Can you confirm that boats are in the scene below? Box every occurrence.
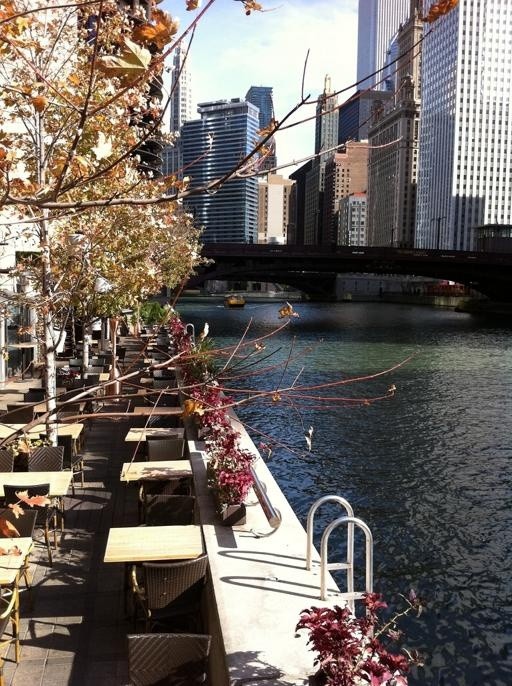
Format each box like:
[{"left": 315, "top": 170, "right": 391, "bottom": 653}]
[{"left": 223, "top": 297, "right": 246, "bottom": 308}]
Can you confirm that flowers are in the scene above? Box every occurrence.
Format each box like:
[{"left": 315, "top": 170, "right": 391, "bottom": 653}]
[
  {"left": 295, "top": 591, "right": 424, "bottom": 686},
  {"left": 193, "top": 381, "right": 257, "bottom": 505}
]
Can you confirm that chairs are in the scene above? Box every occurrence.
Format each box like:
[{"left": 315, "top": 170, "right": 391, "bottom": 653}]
[{"left": 0, "top": 329, "right": 212, "bottom": 686}]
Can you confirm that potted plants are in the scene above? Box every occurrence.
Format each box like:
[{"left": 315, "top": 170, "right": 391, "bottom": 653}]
[{"left": 120, "top": 314, "right": 129, "bottom": 337}]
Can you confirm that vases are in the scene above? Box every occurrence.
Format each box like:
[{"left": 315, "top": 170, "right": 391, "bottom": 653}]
[{"left": 211, "top": 486, "right": 246, "bottom": 525}]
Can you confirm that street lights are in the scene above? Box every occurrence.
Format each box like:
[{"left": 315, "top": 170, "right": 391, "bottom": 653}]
[
  {"left": 384, "top": 224, "right": 401, "bottom": 247},
  {"left": 431, "top": 216, "right": 447, "bottom": 249}
]
